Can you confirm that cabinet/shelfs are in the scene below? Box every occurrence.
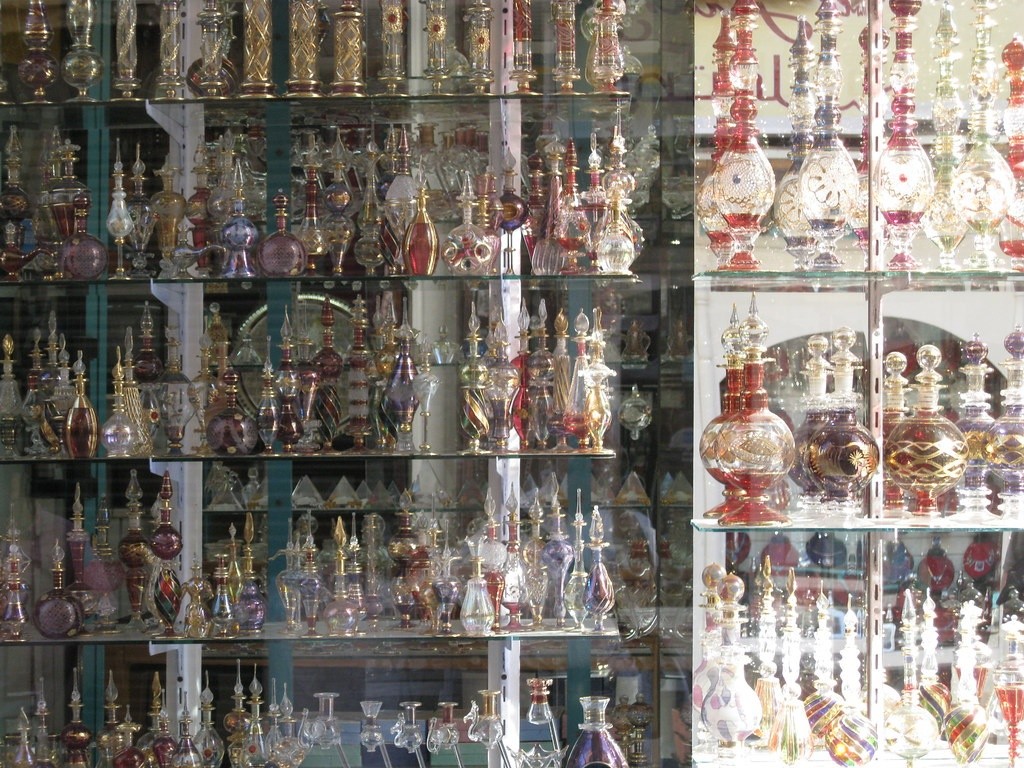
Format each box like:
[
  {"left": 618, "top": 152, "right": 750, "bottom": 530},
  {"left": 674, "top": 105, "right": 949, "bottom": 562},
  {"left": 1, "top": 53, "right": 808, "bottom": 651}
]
[{"left": 0, "top": 0, "right": 1024, "bottom": 768}]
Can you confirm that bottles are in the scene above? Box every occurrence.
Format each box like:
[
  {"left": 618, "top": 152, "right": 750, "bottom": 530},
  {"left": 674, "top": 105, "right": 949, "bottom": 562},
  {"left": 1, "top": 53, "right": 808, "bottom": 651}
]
[{"left": 0, "top": 1, "right": 1024, "bottom": 766}]
